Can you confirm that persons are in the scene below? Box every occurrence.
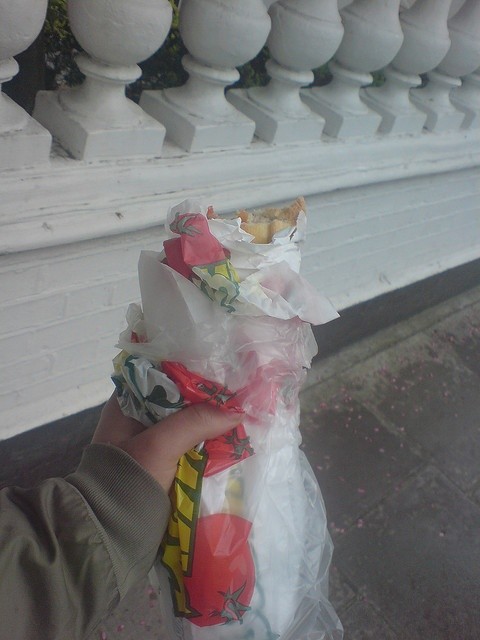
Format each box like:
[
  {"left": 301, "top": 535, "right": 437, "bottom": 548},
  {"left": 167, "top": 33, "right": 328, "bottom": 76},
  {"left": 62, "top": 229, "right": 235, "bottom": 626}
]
[{"left": 1, "top": 387, "right": 247, "bottom": 639}]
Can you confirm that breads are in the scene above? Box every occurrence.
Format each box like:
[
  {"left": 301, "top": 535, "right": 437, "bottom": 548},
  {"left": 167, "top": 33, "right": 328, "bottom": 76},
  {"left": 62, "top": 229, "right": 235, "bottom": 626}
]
[{"left": 207, "top": 194, "right": 308, "bottom": 243}]
